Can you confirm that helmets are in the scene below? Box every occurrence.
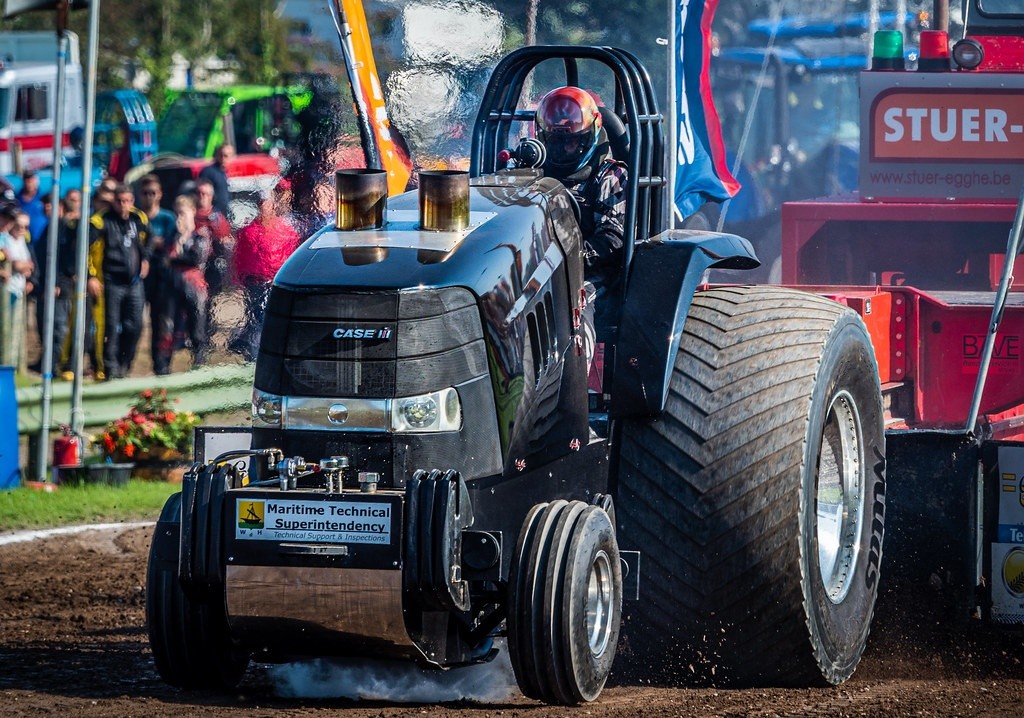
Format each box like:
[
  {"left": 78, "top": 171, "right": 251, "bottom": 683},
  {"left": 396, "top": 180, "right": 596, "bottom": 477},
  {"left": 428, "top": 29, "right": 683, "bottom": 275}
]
[{"left": 534, "top": 86, "right": 602, "bottom": 177}]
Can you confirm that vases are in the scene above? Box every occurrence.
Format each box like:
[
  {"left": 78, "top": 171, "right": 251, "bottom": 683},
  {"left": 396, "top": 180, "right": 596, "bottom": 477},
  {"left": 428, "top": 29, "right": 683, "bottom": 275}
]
[
  {"left": 60, "top": 460, "right": 132, "bottom": 486},
  {"left": 131, "top": 447, "right": 188, "bottom": 483}
]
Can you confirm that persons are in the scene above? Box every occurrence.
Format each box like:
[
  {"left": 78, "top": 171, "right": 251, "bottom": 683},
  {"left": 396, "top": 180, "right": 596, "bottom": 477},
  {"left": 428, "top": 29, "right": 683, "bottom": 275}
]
[
  {"left": 0, "top": 142, "right": 337, "bottom": 382},
  {"left": 535, "top": 86, "right": 629, "bottom": 378}
]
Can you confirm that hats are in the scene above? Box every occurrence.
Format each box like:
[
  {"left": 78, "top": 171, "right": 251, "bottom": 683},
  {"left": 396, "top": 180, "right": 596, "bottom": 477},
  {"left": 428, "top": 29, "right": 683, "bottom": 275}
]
[
  {"left": 114, "top": 185, "right": 133, "bottom": 193},
  {"left": 141, "top": 174, "right": 160, "bottom": 184},
  {"left": 275, "top": 179, "right": 292, "bottom": 194}
]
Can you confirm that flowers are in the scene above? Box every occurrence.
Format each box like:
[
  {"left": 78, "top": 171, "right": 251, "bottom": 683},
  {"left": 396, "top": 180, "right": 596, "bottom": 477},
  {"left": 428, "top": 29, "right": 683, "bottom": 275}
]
[{"left": 95, "top": 390, "right": 201, "bottom": 465}]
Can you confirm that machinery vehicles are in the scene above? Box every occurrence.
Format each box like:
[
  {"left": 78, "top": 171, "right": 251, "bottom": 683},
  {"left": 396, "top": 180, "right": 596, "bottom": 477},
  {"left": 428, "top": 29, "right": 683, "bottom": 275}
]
[
  {"left": 144, "top": 47, "right": 886, "bottom": 709},
  {"left": 0, "top": 1, "right": 1024, "bottom": 450}
]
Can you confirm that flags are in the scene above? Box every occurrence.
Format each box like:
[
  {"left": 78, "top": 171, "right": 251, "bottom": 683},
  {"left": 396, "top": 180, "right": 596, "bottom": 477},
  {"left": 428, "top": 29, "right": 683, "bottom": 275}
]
[{"left": 670, "top": 0, "right": 741, "bottom": 223}]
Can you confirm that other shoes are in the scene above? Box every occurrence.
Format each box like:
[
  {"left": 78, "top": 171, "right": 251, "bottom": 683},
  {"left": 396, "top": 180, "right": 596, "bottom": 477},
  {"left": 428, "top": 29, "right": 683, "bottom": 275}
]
[
  {"left": 96, "top": 371, "right": 105, "bottom": 379},
  {"left": 61, "top": 370, "right": 74, "bottom": 380}
]
